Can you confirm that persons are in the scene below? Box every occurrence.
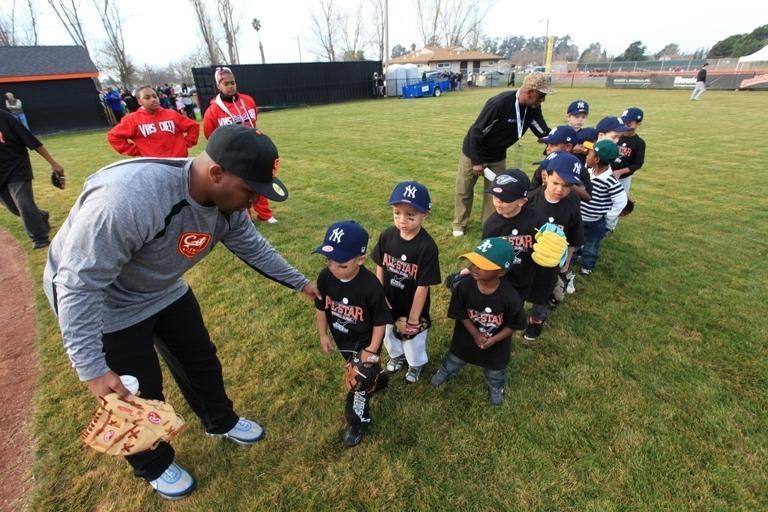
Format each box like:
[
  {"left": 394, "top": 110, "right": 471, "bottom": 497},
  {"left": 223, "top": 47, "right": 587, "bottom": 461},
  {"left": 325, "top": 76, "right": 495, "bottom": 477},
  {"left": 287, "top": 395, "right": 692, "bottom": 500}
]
[
  {"left": 507, "top": 65, "right": 515, "bottom": 87},
  {"left": 376, "top": 75, "right": 384, "bottom": 98},
  {"left": 690, "top": 63, "right": 709, "bottom": 101},
  {"left": 40, "top": 123, "right": 325, "bottom": 503},
  {"left": 572, "top": 126, "right": 598, "bottom": 155},
  {"left": 610, "top": 107, "right": 645, "bottom": 193},
  {"left": 104, "top": 85, "right": 200, "bottom": 158},
  {"left": 445, "top": 169, "right": 570, "bottom": 306},
  {"left": 200, "top": 66, "right": 279, "bottom": 224},
  {"left": 529, "top": 125, "right": 593, "bottom": 203},
  {"left": 428, "top": 238, "right": 529, "bottom": 409},
  {"left": 543, "top": 98, "right": 598, "bottom": 165},
  {"left": 421, "top": 68, "right": 473, "bottom": 92},
  {"left": 4, "top": 92, "right": 30, "bottom": 133},
  {"left": 308, "top": 218, "right": 391, "bottom": 448},
  {"left": 597, "top": 116, "right": 634, "bottom": 144},
  {"left": 0, "top": 108, "right": 67, "bottom": 250},
  {"left": 570, "top": 139, "right": 628, "bottom": 275},
  {"left": 367, "top": 180, "right": 443, "bottom": 386},
  {"left": 451, "top": 71, "right": 561, "bottom": 237},
  {"left": 103, "top": 83, "right": 198, "bottom": 123},
  {"left": 524, "top": 151, "right": 586, "bottom": 342}
]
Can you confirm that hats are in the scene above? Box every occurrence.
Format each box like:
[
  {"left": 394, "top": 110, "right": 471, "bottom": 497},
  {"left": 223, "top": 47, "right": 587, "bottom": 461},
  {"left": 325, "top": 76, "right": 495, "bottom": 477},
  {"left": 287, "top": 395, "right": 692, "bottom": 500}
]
[
  {"left": 457, "top": 237, "right": 516, "bottom": 272},
  {"left": 483, "top": 169, "right": 530, "bottom": 203},
  {"left": 387, "top": 182, "right": 432, "bottom": 212},
  {"left": 523, "top": 71, "right": 558, "bottom": 95},
  {"left": 311, "top": 221, "right": 369, "bottom": 264},
  {"left": 205, "top": 124, "right": 288, "bottom": 201},
  {"left": 533, "top": 100, "right": 643, "bottom": 184}
]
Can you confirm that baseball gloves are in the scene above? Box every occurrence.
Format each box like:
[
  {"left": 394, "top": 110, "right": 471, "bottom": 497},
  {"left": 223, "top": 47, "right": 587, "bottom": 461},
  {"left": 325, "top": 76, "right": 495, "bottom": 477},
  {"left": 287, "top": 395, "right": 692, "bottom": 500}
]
[
  {"left": 532, "top": 223, "right": 568, "bottom": 269},
  {"left": 82, "top": 393, "right": 184, "bottom": 456}
]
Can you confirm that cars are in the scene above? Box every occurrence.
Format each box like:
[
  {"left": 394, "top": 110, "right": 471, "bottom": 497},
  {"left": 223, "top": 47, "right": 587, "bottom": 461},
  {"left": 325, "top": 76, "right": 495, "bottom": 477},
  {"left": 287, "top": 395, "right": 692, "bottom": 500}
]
[{"left": 524, "top": 66, "right": 545, "bottom": 74}]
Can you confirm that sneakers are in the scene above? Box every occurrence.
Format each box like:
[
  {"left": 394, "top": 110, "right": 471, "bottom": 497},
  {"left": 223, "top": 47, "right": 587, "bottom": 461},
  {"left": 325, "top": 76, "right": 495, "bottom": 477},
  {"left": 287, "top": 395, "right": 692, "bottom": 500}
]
[
  {"left": 32, "top": 210, "right": 49, "bottom": 248},
  {"left": 149, "top": 461, "right": 197, "bottom": 501},
  {"left": 452, "top": 230, "right": 464, "bottom": 237},
  {"left": 386, "top": 353, "right": 449, "bottom": 388},
  {"left": 205, "top": 416, "right": 264, "bottom": 444},
  {"left": 267, "top": 216, "right": 278, "bottom": 224},
  {"left": 489, "top": 385, "right": 503, "bottom": 405},
  {"left": 523, "top": 256, "right": 592, "bottom": 341},
  {"left": 344, "top": 423, "right": 368, "bottom": 445}
]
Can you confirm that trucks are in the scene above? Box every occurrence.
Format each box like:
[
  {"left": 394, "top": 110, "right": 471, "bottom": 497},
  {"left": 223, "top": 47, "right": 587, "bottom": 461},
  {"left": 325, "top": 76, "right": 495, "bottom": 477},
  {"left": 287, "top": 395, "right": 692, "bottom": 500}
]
[{"left": 402, "top": 69, "right": 461, "bottom": 99}]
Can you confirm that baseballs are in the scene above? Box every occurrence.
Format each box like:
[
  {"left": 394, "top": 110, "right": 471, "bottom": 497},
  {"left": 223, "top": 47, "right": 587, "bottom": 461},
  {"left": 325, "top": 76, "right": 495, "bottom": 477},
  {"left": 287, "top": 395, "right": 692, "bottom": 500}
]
[{"left": 119, "top": 375, "right": 139, "bottom": 394}]
